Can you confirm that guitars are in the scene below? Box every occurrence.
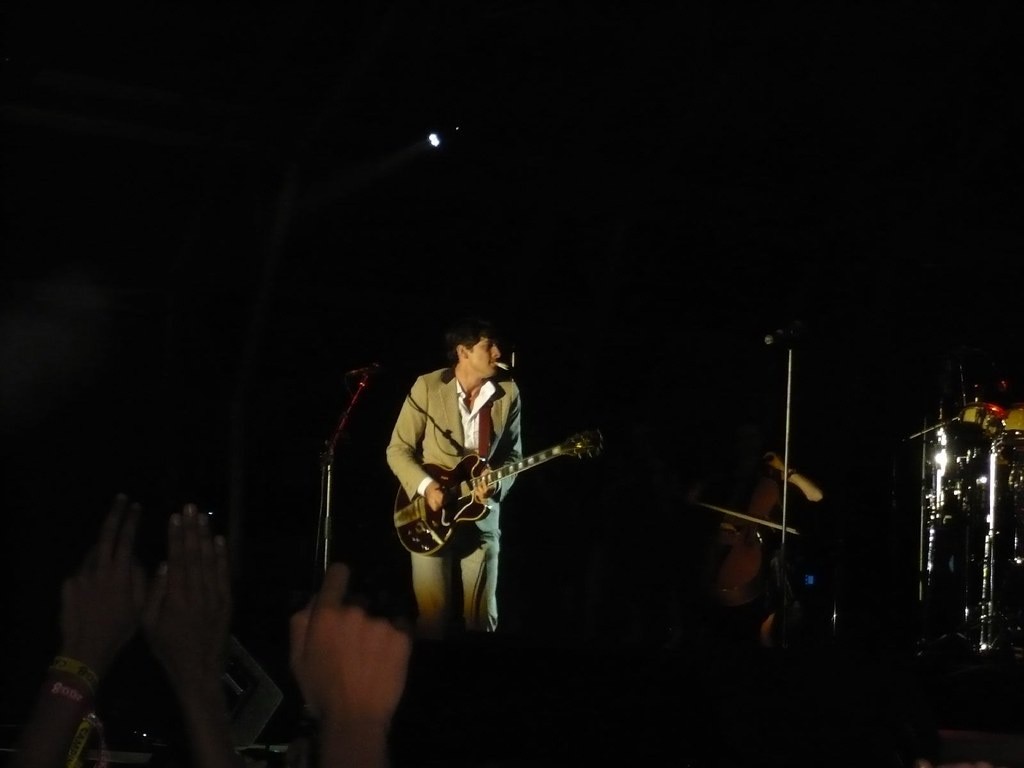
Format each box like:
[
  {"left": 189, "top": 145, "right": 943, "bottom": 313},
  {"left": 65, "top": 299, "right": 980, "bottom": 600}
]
[{"left": 393, "top": 428, "right": 606, "bottom": 557}]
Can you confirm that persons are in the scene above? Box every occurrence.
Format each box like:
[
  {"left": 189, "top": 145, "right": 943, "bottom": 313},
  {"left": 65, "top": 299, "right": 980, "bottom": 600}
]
[
  {"left": 387, "top": 313, "right": 524, "bottom": 635},
  {"left": 16, "top": 489, "right": 413, "bottom": 768},
  {"left": 689, "top": 417, "right": 823, "bottom": 582}
]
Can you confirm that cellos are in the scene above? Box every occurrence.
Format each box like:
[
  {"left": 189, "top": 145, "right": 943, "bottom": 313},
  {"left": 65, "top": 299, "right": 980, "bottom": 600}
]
[{"left": 695, "top": 318, "right": 808, "bottom": 607}]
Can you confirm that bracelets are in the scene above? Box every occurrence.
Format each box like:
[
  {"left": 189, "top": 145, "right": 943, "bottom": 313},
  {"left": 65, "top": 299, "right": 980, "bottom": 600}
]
[
  {"left": 47, "top": 654, "right": 101, "bottom": 693},
  {"left": 36, "top": 680, "right": 87, "bottom": 708},
  {"left": 785, "top": 469, "right": 795, "bottom": 480}
]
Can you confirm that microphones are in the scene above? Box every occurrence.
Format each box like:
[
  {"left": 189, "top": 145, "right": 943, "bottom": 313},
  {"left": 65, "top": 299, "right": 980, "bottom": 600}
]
[
  {"left": 345, "top": 361, "right": 381, "bottom": 377},
  {"left": 764, "top": 318, "right": 804, "bottom": 342}
]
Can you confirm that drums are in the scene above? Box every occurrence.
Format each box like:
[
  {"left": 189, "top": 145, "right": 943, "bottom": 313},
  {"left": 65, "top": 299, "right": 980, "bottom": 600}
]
[
  {"left": 961, "top": 401, "right": 1004, "bottom": 456},
  {"left": 915, "top": 416, "right": 993, "bottom": 531},
  {"left": 1001, "top": 403, "right": 1024, "bottom": 466}
]
